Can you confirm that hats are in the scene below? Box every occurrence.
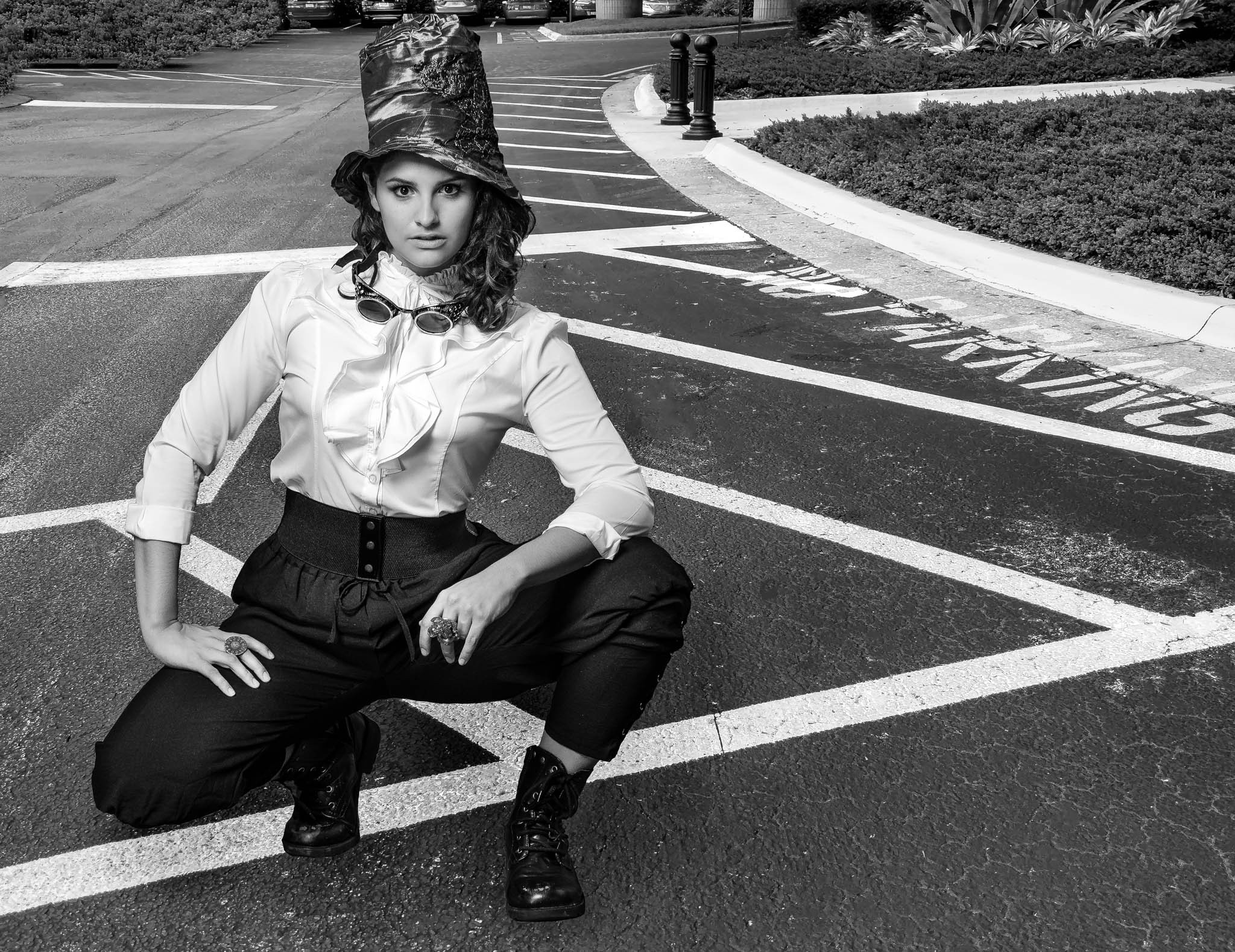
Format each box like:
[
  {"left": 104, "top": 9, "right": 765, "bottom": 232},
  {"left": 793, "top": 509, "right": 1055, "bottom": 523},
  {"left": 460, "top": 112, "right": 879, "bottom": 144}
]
[{"left": 332, "top": 12, "right": 523, "bottom": 205}]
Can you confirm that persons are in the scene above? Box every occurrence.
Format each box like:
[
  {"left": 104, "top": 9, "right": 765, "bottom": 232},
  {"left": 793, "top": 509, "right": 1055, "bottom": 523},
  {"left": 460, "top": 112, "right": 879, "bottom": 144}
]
[{"left": 91, "top": 15, "right": 693, "bottom": 922}]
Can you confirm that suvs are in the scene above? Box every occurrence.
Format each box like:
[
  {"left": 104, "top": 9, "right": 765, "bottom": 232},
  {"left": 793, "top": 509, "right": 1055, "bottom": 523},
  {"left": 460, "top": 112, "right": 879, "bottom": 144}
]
[
  {"left": 431, "top": 0, "right": 482, "bottom": 25},
  {"left": 361, "top": 0, "right": 408, "bottom": 25}
]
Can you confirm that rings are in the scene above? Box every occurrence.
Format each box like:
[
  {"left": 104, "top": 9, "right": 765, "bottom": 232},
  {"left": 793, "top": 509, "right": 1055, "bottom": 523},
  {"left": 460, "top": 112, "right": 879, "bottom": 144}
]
[
  {"left": 427, "top": 617, "right": 461, "bottom": 646},
  {"left": 224, "top": 636, "right": 248, "bottom": 656}
]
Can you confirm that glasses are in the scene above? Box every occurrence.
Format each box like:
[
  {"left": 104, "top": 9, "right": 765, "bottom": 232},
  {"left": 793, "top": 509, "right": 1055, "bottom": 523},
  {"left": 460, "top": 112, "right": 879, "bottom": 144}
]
[{"left": 354, "top": 281, "right": 464, "bottom": 334}]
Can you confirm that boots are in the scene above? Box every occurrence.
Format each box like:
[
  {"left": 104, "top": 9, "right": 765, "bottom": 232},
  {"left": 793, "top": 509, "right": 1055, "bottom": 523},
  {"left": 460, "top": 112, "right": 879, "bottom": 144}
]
[
  {"left": 253, "top": 718, "right": 382, "bottom": 858},
  {"left": 502, "top": 739, "right": 585, "bottom": 922}
]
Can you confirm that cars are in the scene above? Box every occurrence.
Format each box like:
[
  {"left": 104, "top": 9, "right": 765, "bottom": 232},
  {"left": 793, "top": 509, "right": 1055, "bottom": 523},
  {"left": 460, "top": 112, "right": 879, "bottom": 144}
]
[
  {"left": 288, "top": 0, "right": 347, "bottom": 25},
  {"left": 502, "top": 0, "right": 553, "bottom": 25},
  {"left": 565, "top": 0, "right": 596, "bottom": 21},
  {"left": 642, "top": 0, "right": 686, "bottom": 18}
]
